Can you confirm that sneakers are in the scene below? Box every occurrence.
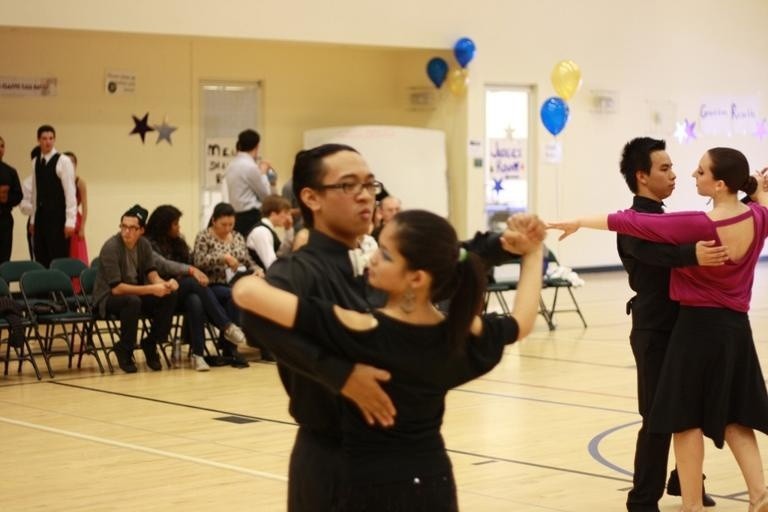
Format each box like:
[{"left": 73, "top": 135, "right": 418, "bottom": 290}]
[
  {"left": 114, "top": 341, "right": 137, "bottom": 372},
  {"left": 140, "top": 337, "right": 161, "bottom": 370},
  {"left": 223, "top": 324, "right": 245, "bottom": 345},
  {"left": 190, "top": 353, "right": 210, "bottom": 371}
]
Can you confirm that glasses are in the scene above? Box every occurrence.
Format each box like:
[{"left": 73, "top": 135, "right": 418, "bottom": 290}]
[
  {"left": 314, "top": 179, "right": 383, "bottom": 196},
  {"left": 119, "top": 223, "right": 141, "bottom": 231}
]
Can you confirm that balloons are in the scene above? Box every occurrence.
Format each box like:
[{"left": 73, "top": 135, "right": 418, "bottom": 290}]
[
  {"left": 427, "top": 56, "right": 447, "bottom": 88},
  {"left": 453, "top": 36, "right": 476, "bottom": 67},
  {"left": 444, "top": 70, "right": 468, "bottom": 103},
  {"left": 266, "top": 167, "right": 278, "bottom": 187},
  {"left": 541, "top": 97, "right": 570, "bottom": 138},
  {"left": 550, "top": 58, "right": 581, "bottom": 104}
]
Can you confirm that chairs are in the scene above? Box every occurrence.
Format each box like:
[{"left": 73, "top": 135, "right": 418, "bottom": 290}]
[
  {"left": 465, "top": 227, "right": 588, "bottom": 332},
  {"left": 0, "top": 256, "right": 227, "bottom": 381}
]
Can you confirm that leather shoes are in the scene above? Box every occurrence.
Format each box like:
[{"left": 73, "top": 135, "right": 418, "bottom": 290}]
[
  {"left": 261, "top": 351, "right": 277, "bottom": 362},
  {"left": 223, "top": 349, "right": 248, "bottom": 365},
  {"left": 666, "top": 479, "right": 715, "bottom": 506}
]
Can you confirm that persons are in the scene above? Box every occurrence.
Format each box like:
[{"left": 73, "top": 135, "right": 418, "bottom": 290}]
[
  {"left": 247, "top": 194, "right": 401, "bottom": 278},
  {"left": 143, "top": 204, "right": 245, "bottom": 371},
  {"left": 225, "top": 127, "right": 273, "bottom": 235},
  {"left": 28, "top": 124, "right": 79, "bottom": 302},
  {"left": 90, "top": 204, "right": 178, "bottom": 374},
  {"left": 281, "top": 177, "right": 305, "bottom": 234},
  {"left": 63, "top": 152, "right": 90, "bottom": 297},
  {"left": 0, "top": 136, "right": 23, "bottom": 262},
  {"left": 546, "top": 146, "right": 768, "bottom": 512},
  {"left": 192, "top": 201, "right": 277, "bottom": 369},
  {"left": 241, "top": 141, "right": 550, "bottom": 512},
  {"left": 609, "top": 137, "right": 768, "bottom": 512},
  {"left": 224, "top": 206, "right": 545, "bottom": 512},
  {"left": 18, "top": 145, "right": 41, "bottom": 261}
]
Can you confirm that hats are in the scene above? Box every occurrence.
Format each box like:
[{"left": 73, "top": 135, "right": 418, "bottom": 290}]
[{"left": 125, "top": 205, "right": 148, "bottom": 225}]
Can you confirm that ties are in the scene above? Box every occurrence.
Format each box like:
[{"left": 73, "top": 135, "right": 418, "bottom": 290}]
[{"left": 41, "top": 158, "right": 45, "bottom": 165}]
